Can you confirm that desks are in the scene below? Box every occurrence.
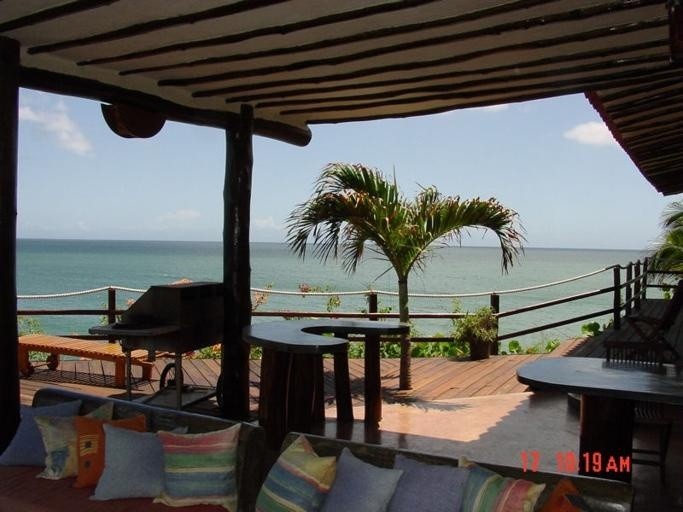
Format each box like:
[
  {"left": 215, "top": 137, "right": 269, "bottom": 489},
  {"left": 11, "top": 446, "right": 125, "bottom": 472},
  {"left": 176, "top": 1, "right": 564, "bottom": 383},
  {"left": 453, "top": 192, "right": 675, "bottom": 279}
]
[
  {"left": 240, "top": 309, "right": 412, "bottom": 438},
  {"left": 516, "top": 352, "right": 680, "bottom": 489}
]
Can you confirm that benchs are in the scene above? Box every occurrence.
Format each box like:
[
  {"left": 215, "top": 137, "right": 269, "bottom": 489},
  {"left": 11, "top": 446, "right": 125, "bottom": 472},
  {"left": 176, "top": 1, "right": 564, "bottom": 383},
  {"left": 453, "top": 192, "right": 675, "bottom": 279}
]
[{"left": 17, "top": 332, "right": 160, "bottom": 390}]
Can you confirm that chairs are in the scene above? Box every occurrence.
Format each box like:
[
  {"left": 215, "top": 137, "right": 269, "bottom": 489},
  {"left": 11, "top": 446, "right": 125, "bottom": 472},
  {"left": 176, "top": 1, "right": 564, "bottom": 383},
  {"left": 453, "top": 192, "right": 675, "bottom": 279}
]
[{"left": 619, "top": 278, "right": 681, "bottom": 364}]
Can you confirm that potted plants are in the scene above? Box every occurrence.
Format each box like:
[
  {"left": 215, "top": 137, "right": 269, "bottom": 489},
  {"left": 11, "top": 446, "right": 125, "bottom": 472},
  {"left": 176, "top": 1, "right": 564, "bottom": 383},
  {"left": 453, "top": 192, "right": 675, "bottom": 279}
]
[{"left": 449, "top": 304, "right": 497, "bottom": 361}]
